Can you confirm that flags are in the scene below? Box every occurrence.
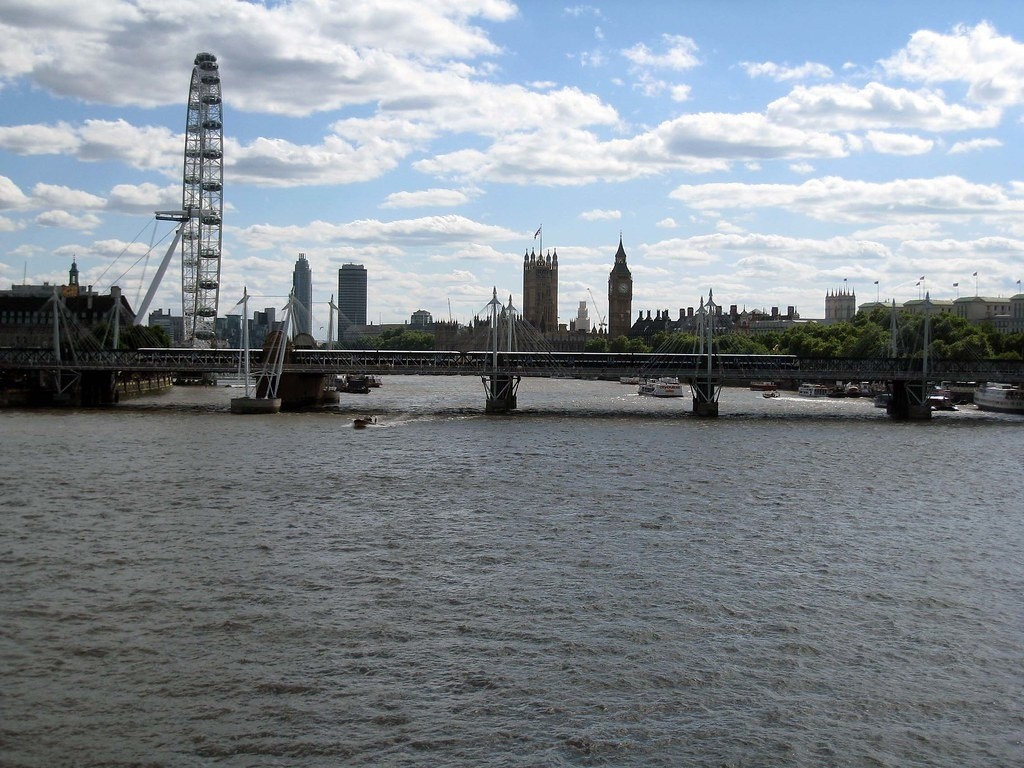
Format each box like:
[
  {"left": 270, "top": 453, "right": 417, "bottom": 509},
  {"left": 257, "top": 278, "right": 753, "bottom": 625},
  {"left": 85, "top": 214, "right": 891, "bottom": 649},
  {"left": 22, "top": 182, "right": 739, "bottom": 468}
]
[
  {"left": 920, "top": 276, "right": 924, "bottom": 280},
  {"left": 953, "top": 283, "right": 958, "bottom": 287},
  {"left": 844, "top": 278, "right": 847, "bottom": 281},
  {"left": 1017, "top": 280, "right": 1020, "bottom": 284},
  {"left": 534, "top": 227, "right": 541, "bottom": 239},
  {"left": 916, "top": 282, "right": 920, "bottom": 286},
  {"left": 973, "top": 272, "right": 977, "bottom": 276},
  {"left": 874, "top": 281, "right": 879, "bottom": 284}
]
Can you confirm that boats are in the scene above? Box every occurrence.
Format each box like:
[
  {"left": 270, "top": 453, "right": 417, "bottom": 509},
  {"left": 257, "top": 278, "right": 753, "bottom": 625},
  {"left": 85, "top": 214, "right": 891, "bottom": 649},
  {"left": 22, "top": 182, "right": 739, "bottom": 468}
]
[
  {"left": 798, "top": 383, "right": 828, "bottom": 398},
  {"left": 972, "top": 381, "right": 1024, "bottom": 413},
  {"left": 620, "top": 377, "right": 638, "bottom": 384},
  {"left": 763, "top": 391, "right": 780, "bottom": 399},
  {"left": 638, "top": 377, "right": 682, "bottom": 398},
  {"left": 750, "top": 381, "right": 776, "bottom": 392},
  {"left": 354, "top": 416, "right": 378, "bottom": 429},
  {"left": 873, "top": 395, "right": 958, "bottom": 411},
  {"left": 941, "top": 381, "right": 978, "bottom": 400},
  {"left": 828, "top": 387, "right": 846, "bottom": 398},
  {"left": 845, "top": 387, "right": 861, "bottom": 397},
  {"left": 333, "top": 373, "right": 383, "bottom": 394}
]
